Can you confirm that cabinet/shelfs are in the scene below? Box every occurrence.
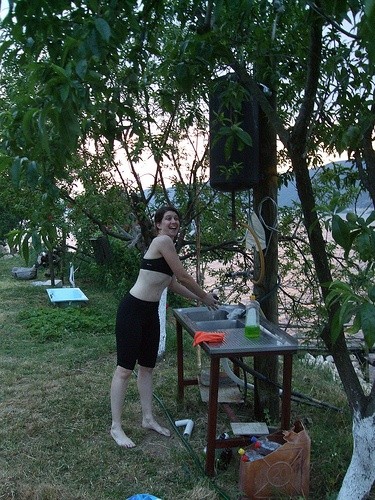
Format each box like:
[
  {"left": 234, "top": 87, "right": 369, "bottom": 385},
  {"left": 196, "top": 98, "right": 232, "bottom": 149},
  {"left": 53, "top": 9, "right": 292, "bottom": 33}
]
[{"left": 170, "top": 305, "right": 300, "bottom": 477}]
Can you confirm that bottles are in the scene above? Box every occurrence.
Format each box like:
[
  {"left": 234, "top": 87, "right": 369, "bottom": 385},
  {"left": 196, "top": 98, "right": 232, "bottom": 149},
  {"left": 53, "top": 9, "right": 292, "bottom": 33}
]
[
  {"left": 245, "top": 294, "right": 262, "bottom": 338},
  {"left": 204, "top": 433, "right": 231, "bottom": 472},
  {"left": 237, "top": 437, "right": 282, "bottom": 463}
]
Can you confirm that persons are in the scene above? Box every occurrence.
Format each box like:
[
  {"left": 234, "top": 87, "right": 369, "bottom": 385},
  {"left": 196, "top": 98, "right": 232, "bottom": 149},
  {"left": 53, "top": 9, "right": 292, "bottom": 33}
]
[
  {"left": 110, "top": 206, "right": 224, "bottom": 449},
  {"left": 0, "top": 241, "right": 61, "bottom": 278}
]
